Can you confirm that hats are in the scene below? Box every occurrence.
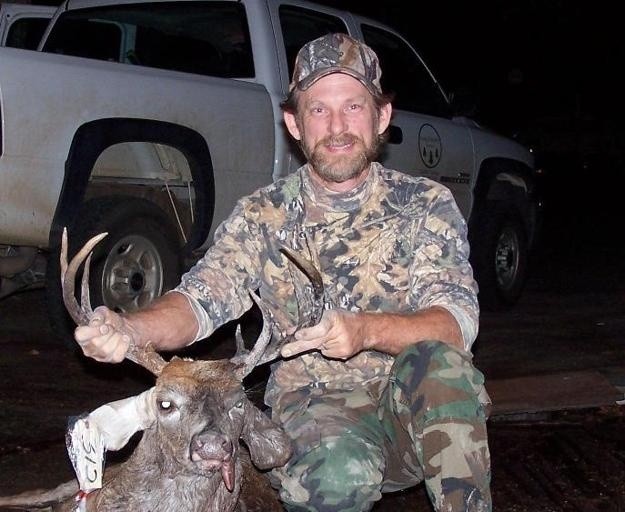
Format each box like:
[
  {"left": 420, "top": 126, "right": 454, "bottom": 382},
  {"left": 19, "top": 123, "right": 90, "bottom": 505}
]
[{"left": 289, "top": 34, "right": 382, "bottom": 97}]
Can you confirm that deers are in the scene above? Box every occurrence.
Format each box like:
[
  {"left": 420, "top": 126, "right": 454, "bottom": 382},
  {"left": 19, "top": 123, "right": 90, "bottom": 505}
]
[{"left": 0, "top": 227, "right": 327, "bottom": 511}]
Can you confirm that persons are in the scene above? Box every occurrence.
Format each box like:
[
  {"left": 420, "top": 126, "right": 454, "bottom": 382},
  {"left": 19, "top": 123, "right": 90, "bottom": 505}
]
[{"left": 74, "top": 33, "right": 495, "bottom": 512}]
